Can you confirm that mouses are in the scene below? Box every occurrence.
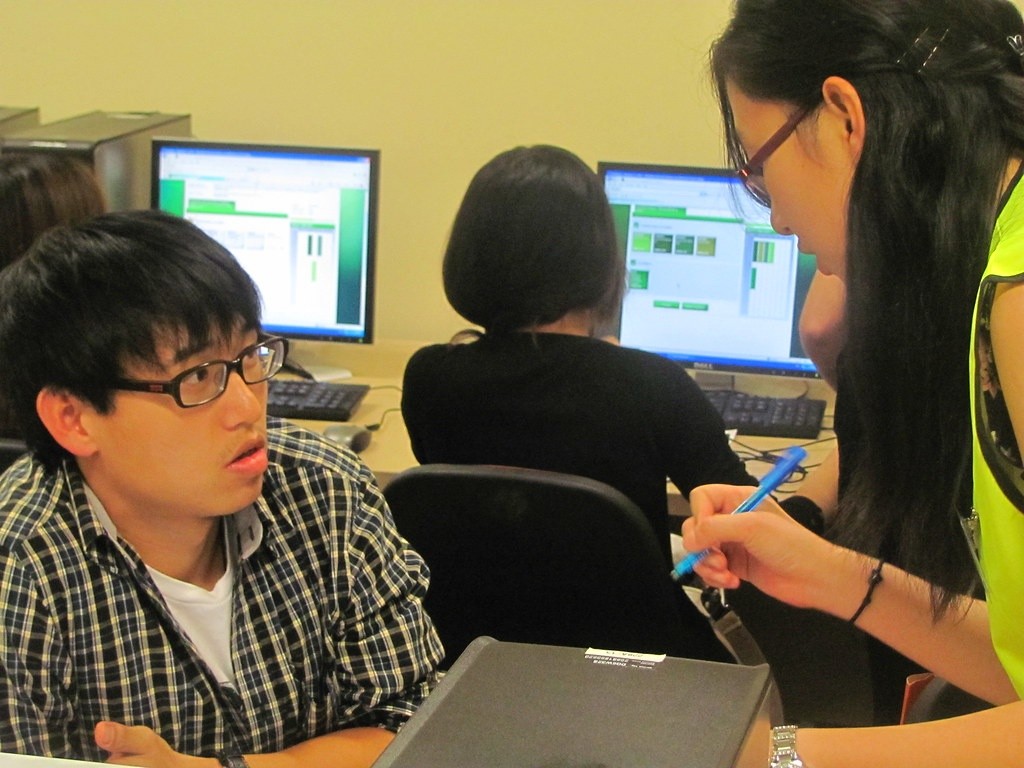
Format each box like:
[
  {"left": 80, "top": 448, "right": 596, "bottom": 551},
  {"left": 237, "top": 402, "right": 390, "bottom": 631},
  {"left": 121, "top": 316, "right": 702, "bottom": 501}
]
[{"left": 323, "top": 426, "right": 372, "bottom": 454}]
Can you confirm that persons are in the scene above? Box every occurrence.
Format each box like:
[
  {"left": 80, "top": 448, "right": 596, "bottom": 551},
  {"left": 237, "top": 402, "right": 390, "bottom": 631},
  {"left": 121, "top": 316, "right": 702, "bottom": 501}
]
[
  {"left": 683, "top": 0, "right": 1024, "bottom": 768},
  {"left": 0, "top": 211, "right": 447, "bottom": 768},
  {"left": 402, "top": 145, "right": 840, "bottom": 577},
  {"left": 0, "top": 153, "right": 105, "bottom": 273}
]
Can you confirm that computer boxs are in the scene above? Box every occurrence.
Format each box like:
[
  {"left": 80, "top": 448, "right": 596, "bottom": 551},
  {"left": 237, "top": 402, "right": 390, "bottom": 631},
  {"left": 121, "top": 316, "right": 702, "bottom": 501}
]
[{"left": 0, "top": 104, "right": 191, "bottom": 214}]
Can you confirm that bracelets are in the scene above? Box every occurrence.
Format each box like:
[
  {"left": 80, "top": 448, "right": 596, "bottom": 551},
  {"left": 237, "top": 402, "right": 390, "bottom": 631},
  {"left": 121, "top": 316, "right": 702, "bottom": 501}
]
[
  {"left": 839, "top": 555, "right": 885, "bottom": 624},
  {"left": 200, "top": 748, "right": 249, "bottom": 768}
]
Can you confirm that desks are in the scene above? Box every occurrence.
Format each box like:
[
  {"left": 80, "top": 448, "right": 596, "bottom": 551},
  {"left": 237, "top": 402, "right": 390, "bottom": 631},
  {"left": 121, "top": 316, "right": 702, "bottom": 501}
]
[{"left": 265, "top": 378, "right": 840, "bottom": 530}]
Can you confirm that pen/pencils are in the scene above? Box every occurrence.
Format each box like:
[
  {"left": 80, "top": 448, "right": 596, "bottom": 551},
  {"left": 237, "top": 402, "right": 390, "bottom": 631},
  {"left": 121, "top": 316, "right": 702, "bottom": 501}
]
[{"left": 670, "top": 444, "right": 806, "bottom": 582}]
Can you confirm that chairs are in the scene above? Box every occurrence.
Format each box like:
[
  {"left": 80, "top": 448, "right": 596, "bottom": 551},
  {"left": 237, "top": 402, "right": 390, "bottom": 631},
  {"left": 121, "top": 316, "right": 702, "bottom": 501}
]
[{"left": 380, "top": 464, "right": 732, "bottom": 671}]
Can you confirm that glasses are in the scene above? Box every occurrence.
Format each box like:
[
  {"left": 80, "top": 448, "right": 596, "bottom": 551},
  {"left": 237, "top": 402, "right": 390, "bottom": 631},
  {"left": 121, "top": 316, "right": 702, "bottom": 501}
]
[
  {"left": 103, "top": 332, "right": 289, "bottom": 409},
  {"left": 735, "top": 95, "right": 823, "bottom": 209}
]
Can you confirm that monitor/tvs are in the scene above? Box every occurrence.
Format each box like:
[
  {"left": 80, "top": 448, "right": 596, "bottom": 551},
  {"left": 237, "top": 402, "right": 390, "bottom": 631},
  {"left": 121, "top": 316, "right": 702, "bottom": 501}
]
[
  {"left": 148, "top": 137, "right": 381, "bottom": 380},
  {"left": 598, "top": 161, "right": 824, "bottom": 399}
]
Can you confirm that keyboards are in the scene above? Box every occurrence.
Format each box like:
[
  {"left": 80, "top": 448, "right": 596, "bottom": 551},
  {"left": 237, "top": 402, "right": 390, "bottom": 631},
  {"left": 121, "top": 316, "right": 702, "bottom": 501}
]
[
  {"left": 701, "top": 387, "right": 827, "bottom": 442},
  {"left": 266, "top": 379, "right": 368, "bottom": 423}
]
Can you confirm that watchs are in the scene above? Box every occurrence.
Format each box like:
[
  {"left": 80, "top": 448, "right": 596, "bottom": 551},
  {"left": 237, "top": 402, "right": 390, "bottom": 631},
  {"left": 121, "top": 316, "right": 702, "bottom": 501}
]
[{"left": 770, "top": 725, "right": 803, "bottom": 768}]
